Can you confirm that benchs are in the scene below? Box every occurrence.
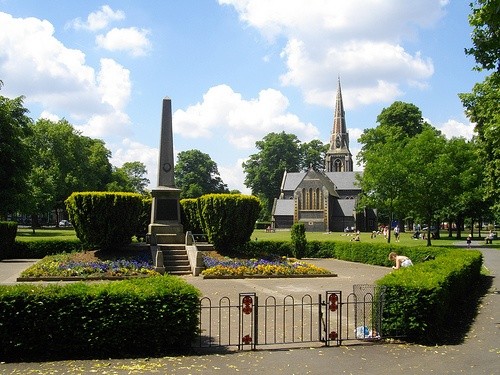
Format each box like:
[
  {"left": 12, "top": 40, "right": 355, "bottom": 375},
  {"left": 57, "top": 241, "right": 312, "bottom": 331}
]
[{"left": 421, "top": 254, "right": 436, "bottom": 262}]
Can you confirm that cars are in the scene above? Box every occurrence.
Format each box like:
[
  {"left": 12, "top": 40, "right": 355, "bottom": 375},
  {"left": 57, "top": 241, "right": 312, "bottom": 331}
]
[{"left": 59, "top": 220, "right": 69, "bottom": 226}]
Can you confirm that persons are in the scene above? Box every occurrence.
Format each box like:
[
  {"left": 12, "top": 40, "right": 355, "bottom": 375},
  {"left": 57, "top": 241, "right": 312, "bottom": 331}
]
[
  {"left": 394, "top": 225, "right": 400, "bottom": 242},
  {"left": 422, "top": 233, "right": 427, "bottom": 239},
  {"left": 383, "top": 226, "right": 388, "bottom": 239},
  {"left": 467, "top": 237, "right": 471, "bottom": 248},
  {"left": 412, "top": 231, "right": 421, "bottom": 239},
  {"left": 388, "top": 252, "right": 414, "bottom": 270},
  {"left": 355, "top": 230, "right": 360, "bottom": 241},
  {"left": 485, "top": 230, "right": 494, "bottom": 244},
  {"left": 430, "top": 233, "right": 435, "bottom": 239}
]
